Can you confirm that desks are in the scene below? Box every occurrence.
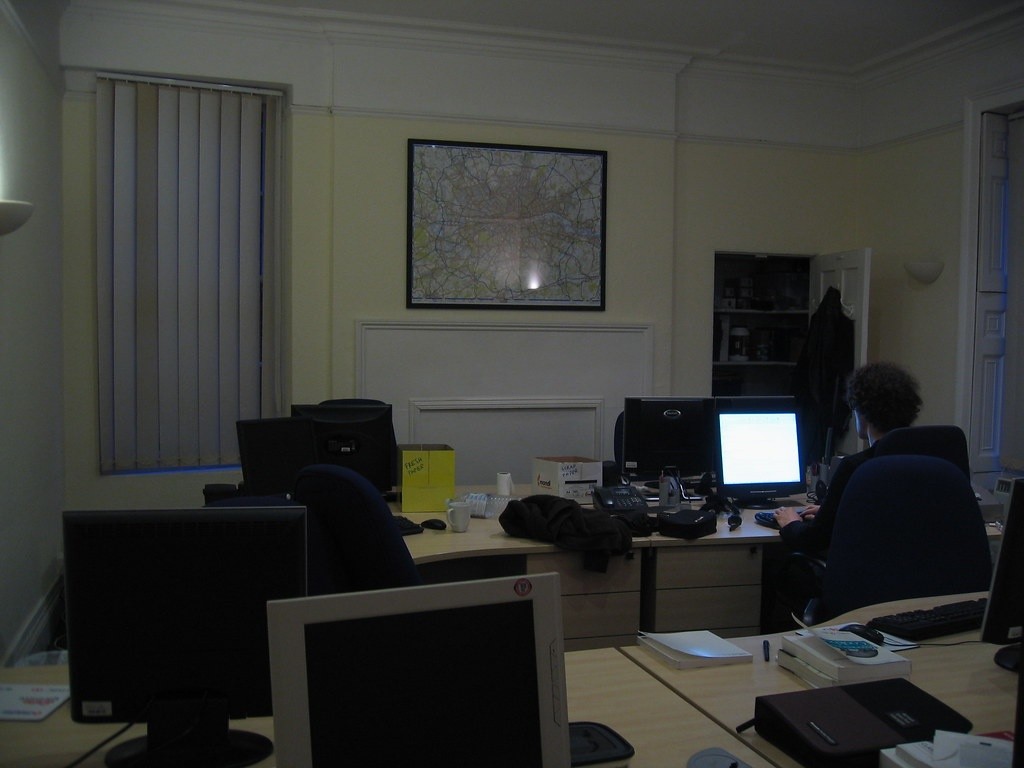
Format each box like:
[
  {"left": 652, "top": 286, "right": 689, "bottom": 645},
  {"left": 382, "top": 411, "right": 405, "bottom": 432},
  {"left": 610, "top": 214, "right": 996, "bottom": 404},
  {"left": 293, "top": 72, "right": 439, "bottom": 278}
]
[{"left": 0, "top": 479, "right": 1021, "bottom": 768}]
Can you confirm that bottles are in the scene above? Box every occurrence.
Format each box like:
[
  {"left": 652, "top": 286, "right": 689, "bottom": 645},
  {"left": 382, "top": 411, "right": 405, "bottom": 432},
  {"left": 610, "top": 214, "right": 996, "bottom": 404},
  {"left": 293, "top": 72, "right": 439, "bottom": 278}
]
[
  {"left": 448, "top": 494, "right": 524, "bottom": 518},
  {"left": 728, "top": 320, "right": 752, "bottom": 362}
]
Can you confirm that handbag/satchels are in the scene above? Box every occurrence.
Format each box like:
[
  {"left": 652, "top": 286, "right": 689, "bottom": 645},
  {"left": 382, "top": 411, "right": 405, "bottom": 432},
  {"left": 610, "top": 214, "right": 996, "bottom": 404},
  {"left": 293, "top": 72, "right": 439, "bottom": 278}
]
[{"left": 659, "top": 509, "right": 717, "bottom": 539}]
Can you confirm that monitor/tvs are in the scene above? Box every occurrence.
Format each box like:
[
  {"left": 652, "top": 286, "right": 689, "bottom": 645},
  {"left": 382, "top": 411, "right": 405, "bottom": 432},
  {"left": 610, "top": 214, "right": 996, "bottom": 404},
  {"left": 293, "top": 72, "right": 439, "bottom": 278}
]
[
  {"left": 623, "top": 396, "right": 807, "bottom": 510},
  {"left": 61, "top": 503, "right": 572, "bottom": 768},
  {"left": 983, "top": 478, "right": 1024, "bottom": 672},
  {"left": 235, "top": 405, "right": 399, "bottom": 496}
]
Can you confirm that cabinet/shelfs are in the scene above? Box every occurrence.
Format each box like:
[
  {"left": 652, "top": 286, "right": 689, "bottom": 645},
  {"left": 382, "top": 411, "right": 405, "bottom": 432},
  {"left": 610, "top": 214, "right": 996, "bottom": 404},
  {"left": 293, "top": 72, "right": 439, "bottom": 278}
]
[{"left": 712, "top": 246, "right": 874, "bottom": 398}]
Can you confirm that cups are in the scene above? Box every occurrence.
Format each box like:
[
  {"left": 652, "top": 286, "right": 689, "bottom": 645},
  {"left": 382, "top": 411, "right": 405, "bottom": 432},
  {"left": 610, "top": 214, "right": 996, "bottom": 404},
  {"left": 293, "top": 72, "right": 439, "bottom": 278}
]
[{"left": 447, "top": 502, "right": 472, "bottom": 532}]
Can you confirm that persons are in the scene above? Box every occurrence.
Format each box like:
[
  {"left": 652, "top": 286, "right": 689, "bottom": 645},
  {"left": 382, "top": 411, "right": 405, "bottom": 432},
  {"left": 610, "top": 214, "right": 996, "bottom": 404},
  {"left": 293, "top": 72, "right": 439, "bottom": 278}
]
[{"left": 771, "top": 360, "right": 925, "bottom": 617}]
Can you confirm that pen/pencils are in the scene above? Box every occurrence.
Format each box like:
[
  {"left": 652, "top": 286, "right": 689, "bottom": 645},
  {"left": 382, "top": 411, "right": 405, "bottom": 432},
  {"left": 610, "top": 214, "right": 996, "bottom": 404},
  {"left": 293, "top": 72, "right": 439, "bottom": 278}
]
[
  {"left": 763, "top": 639, "right": 771, "bottom": 662},
  {"left": 811, "top": 462, "right": 820, "bottom": 475}
]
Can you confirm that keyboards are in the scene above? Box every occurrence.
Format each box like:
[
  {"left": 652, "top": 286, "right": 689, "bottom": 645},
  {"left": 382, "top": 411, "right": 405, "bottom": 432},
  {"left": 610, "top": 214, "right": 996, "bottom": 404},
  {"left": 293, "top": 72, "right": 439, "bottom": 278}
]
[
  {"left": 644, "top": 479, "right": 717, "bottom": 490},
  {"left": 754, "top": 510, "right": 804, "bottom": 530},
  {"left": 393, "top": 515, "right": 424, "bottom": 536},
  {"left": 864, "top": 597, "right": 988, "bottom": 641}
]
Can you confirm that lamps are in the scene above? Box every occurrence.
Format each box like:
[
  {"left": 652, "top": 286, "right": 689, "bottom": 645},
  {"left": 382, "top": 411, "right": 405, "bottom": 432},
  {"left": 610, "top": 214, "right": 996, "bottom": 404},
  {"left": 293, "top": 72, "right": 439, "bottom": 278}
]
[{"left": 903, "top": 260, "right": 944, "bottom": 284}]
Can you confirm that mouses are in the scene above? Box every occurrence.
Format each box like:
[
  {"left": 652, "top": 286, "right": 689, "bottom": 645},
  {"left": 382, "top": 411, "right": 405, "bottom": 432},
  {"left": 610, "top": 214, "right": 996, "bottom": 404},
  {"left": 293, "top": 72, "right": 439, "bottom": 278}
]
[
  {"left": 421, "top": 519, "right": 446, "bottom": 530},
  {"left": 839, "top": 623, "right": 884, "bottom": 644}
]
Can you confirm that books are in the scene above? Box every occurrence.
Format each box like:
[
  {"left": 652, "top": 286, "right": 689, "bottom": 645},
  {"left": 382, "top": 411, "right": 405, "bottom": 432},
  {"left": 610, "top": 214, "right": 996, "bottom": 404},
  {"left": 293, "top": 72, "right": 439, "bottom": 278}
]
[
  {"left": 777, "top": 631, "right": 913, "bottom": 690},
  {"left": 0, "top": 684, "right": 71, "bottom": 722},
  {"left": 879, "top": 731, "right": 1015, "bottom": 768},
  {"left": 636, "top": 630, "right": 753, "bottom": 671},
  {"left": 795, "top": 622, "right": 920, "bottom": 653}
]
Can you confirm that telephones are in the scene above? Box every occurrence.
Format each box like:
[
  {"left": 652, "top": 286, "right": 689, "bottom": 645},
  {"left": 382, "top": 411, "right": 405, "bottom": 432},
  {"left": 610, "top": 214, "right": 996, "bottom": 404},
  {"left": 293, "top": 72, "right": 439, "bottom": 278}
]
[{"left": 591, "top": 486, "right": 649, "bottom": 515}]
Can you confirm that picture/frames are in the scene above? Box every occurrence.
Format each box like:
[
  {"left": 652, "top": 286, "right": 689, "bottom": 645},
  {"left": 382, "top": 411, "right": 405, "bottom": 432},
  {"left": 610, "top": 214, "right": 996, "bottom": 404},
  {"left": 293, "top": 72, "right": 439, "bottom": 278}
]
[{"left": 406, "top": 136, "right": 606, "bottom": 311}]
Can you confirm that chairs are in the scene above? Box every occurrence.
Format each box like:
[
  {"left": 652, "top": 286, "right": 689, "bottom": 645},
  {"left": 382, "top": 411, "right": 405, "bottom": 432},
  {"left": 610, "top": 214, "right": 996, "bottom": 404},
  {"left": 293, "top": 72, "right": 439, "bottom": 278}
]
[{"left": 764, "top": 455, "right": 993, "bottom": 637}]
